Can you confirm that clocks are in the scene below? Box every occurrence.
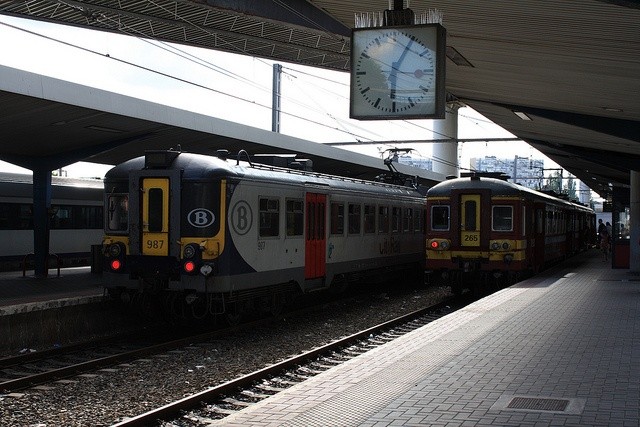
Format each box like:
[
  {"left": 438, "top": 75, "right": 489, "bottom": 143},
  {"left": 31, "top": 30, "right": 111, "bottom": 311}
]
[{"left": 349, "top": 22, "right": 447, "bottom": 121}]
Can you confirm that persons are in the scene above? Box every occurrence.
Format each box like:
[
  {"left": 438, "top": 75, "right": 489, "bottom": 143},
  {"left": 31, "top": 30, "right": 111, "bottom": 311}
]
[
  {"left": 615, "top": 222, "right": 620, "bottom": 239},
  {"left": 600, "top": 231, "right": 609, "bottom": 263},
  {"left": 594, "top": 219, "right": 605, "bottom": 248},
  {"left": 620, "top": 223, "right": 627, "bottom": 239},
  {"left": 605, "top": 222, "right": 612, "bottom": 252}
]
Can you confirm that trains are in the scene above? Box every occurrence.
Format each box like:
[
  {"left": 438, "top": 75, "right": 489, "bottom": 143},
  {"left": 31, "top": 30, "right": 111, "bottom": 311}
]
[
  {"left": 1, "top": 170, "right": 105, "bottom": 274},
  {"left": 102, "top": 146, "right": 427, "bottom": 331},
  {"left": 426, "top": 169, "right": 596, "bottom": 294}
]
